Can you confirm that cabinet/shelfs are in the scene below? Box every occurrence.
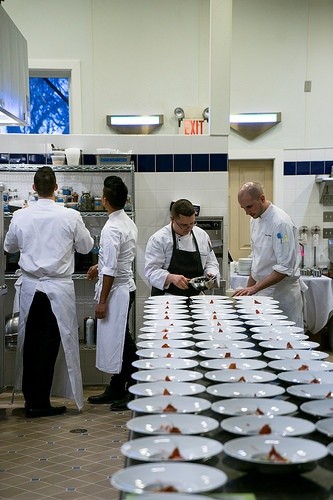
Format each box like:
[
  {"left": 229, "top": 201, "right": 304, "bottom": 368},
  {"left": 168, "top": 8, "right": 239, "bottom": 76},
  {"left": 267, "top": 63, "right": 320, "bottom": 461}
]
[
  {"left": 0, "top": 2, "right": 30, "bottom": 127},
  {"left": 0, "top": 161, "right": 135, "bottom": 392}
]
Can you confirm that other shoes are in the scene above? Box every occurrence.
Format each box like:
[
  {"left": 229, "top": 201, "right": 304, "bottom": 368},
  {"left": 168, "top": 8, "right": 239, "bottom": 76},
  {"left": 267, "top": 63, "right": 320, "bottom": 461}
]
[{"left": 25, "top": 404, "right": 66, "bottom": 417}]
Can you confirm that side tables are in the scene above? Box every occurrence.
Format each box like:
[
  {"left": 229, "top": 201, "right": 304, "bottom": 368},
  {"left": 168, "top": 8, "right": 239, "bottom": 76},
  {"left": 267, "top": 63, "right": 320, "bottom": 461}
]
[{"left": 170, "top": 216, "right": 226, "bottom": 294}]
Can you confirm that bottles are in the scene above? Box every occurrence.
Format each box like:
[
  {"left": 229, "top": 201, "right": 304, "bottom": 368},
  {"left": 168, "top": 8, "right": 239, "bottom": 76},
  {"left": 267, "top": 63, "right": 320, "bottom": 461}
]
[
  {"left": 72, "top": 192, "right": 79, "bottom": 202},
  {"left": 81, "top": 191, "right": 92, "bottom": 211}
]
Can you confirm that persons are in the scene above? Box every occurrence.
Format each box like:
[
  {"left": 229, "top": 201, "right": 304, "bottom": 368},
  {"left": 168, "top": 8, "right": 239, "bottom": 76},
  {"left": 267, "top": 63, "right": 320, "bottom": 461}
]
[
  {"left": 144, "top": 199, "right": 220, "bottom": 296},
  {"left": 87, "top": 175, "right": 139, "bottom": 411},
  {"left": 3, "top": 166, "right": 95, "bottom": 417},
  {"left": 230, "top": 181, "right": 309, "bottom": 333}
]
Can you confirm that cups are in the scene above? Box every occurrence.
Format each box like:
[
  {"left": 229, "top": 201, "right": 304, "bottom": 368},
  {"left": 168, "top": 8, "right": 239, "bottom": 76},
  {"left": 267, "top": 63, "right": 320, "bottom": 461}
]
[{"left": 316, "top": 271, "right": 321, "bottom": 277}]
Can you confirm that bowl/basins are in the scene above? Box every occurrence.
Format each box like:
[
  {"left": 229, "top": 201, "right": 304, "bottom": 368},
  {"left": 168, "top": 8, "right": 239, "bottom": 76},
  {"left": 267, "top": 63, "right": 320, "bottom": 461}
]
[
  {"left": 127, "top": 396, "right": 211, "bottom": 414},
  {"left": 316, "top": 417, "right": 333, "bottom": 437},
  {"left": 211, "top": 398, "right": 298, "bottom": 415},
  {"left": 286, "top": 384, "right": 333, "bottom": 400},
  {"left": 131, "top": 369, "right": 203, "bottom": 381},
  {"left": 53, "top": 151, "right": 65, "bottom": 156},
  {"left": 129, "top": 381, "right": 206, "bottom": 396},
  {"left": 132, "top": 295, "right": 333, "bottom": 371},
  {"left": 126, "top": 414, "right": 220, "bottom": 436},
  {"left": 205, "top": 370, "right": 277, "bottom": 383},
  {"left": 122, "top": 494, "right": 218, "bottom": 500},
  {"left": 51, "top": 156, "right": 65, "bottom": 166},
  {"left": 121, "top": 436, "right": 224, "bottom": 462},
  {"left": 224, "top": 437, "right": 330, "bottom": 464},
  {"left": 221, "top": 416, "right": 317, "bottom": 436},
  {"left": 300, "top": 400, "right": 333, "bottom": 417},
  {"left": 277, "top": 371, "right": 333, "bottom": 384},
  {"left": 111, "top": 462, "right": 227, "bottom": 493},
  {"left": 189, "top": 276, "right": 216, "bottom": 290},
  {"left": 206, "top": 383, "right": 286, "bottom": 398}
]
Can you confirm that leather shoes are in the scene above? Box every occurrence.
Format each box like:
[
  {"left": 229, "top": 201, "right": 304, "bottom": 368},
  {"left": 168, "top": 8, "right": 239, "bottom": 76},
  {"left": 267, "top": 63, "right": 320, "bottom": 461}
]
[
  {"left": 87, "top": 387, "right": 125, "bottom": 404},
  {"left": 110, "top": 393, "right": 135, "bottom": 411}
]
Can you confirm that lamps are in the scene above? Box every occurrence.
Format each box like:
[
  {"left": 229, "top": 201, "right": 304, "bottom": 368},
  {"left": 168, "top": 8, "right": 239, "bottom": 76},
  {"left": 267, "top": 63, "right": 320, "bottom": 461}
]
[
  {"left": 174, "top": 107, "right": 209, "bottom": 134},
  {"left": 106, "top": 114, "right": 164, "bottom": 134},
  {"left": 230, "top": 112, "right": 281, "bottom": 141}
]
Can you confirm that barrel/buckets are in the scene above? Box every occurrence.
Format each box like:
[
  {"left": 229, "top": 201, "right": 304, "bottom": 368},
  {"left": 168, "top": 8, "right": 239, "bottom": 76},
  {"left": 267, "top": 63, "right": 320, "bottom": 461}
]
[{"left": 66, "top": 148, "right": 80, "bottom": 166}]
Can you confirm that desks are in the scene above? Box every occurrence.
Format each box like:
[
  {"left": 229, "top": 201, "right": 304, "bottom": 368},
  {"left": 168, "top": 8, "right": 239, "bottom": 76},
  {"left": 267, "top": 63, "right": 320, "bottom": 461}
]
[
  {"left": 229, "top": 261, "right": 333, "bottom": 350},
  {"left": 118, "top": 307, "right": 333, "bottom": 500}
]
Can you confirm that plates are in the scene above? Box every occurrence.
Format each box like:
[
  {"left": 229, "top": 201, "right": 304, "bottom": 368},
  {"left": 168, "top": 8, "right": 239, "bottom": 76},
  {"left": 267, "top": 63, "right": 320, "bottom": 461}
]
[{"left": 238, "top": 258, "right": 252, "bottom": 276}]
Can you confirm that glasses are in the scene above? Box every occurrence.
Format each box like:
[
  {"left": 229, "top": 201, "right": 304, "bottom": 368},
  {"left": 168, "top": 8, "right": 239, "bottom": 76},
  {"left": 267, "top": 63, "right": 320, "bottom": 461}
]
[{"left": 174, "top": 219, "right": 197, "bottom": 231}]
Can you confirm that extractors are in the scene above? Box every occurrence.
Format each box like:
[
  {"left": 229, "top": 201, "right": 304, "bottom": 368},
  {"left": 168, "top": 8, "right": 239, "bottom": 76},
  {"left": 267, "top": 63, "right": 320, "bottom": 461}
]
[{"left": 0, "top": 5, "right": 30, "bottom": 126}]
